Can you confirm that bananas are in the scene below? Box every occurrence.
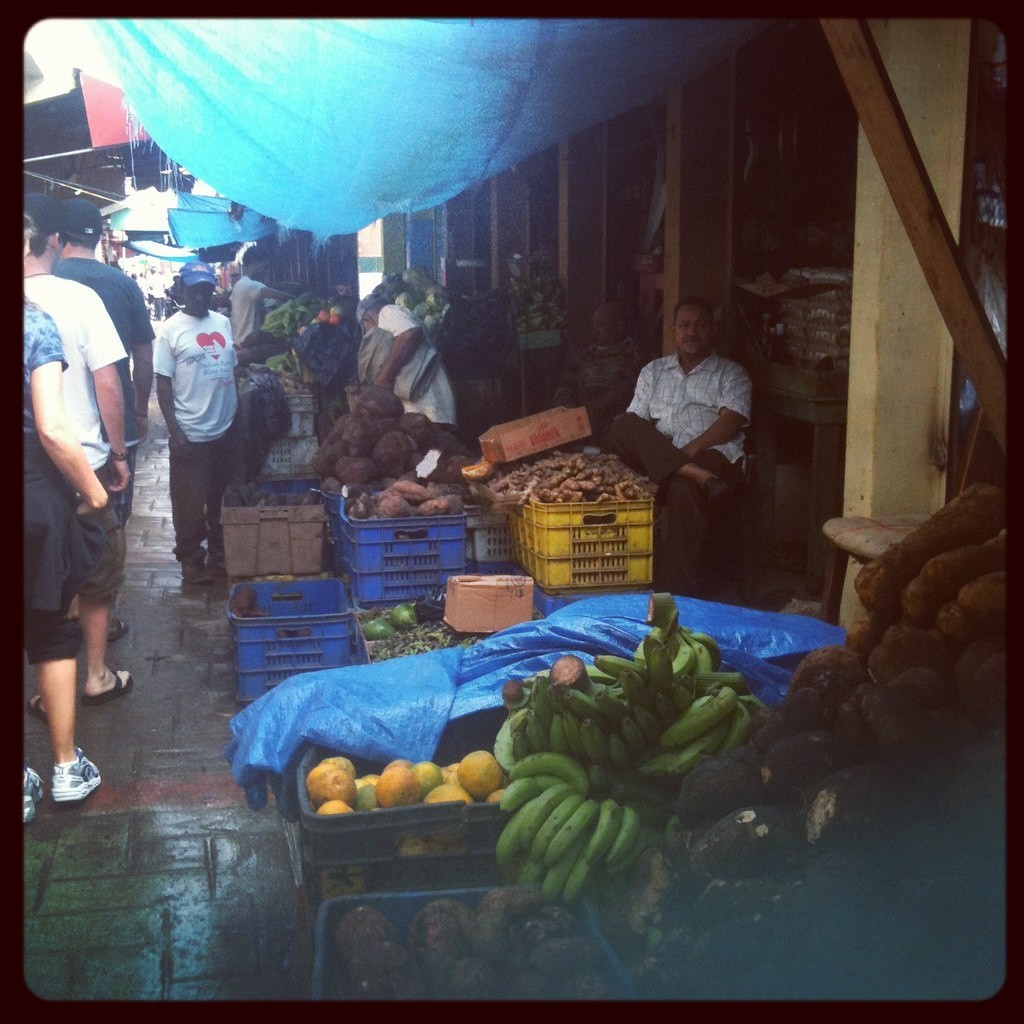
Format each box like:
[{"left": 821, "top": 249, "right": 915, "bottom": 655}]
[{"left": 494, "top": 593, "right": 766, "bottom": 903}]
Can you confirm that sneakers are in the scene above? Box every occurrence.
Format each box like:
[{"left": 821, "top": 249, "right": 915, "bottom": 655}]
[
  {"left": 23, "top": 766, "right": 44, "bottom": 825},
  {"left": 51, "top": 746, "right": 102, "bottom": 802}
]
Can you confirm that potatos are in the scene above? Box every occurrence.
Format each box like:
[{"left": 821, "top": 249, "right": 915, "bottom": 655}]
[
  {"left": 225, "top": 388, "right": 476, "bottom": 638},
  {"left": 332, "top": 886, "right": 610, "bottom": 1000},
  {"left": 600, "top": 482, "right": 1006, "bottom": 978}
]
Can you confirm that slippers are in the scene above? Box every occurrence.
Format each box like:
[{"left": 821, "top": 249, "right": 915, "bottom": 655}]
[
  {"left": 107, "top": 618, "right": 129, "bottom": 642},
  {"left": 28, "top": 694, "right": 48, "bottom": 721},
  {"left": 84, "top": 668, "right": 134, "bottom": 705}
]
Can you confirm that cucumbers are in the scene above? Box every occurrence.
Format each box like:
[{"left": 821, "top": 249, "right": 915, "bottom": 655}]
[{"left": 505, "top": 277, "right": 561, "bottom": 333}]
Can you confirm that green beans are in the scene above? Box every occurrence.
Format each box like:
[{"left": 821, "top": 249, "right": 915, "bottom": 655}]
[{"left": 357, "top": 604, "right": 484, "bottom": 662}]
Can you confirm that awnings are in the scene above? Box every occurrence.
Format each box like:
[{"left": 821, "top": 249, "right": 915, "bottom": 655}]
[{"left": 81, "top": 19, "right": 823, "bottom": 268}]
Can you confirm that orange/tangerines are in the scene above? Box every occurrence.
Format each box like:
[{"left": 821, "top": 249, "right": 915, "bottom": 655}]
[{"left": 309, "top": 750, "right": 505, "bottom": 855}]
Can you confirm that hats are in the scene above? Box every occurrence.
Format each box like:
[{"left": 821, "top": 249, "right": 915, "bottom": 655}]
[
  {"left": 63, "top": 198, "right": 103, "bottom": 237},
  {"left": 179, "top": 262, "right": 216, "bottom": 287},
  {"left": 24, "top": 192, "right": 69, "bottom": 234}
]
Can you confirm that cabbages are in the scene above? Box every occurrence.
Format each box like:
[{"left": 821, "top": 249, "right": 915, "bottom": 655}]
[{"left": 394, "top": 269, "right": 450, "bottom": 331}]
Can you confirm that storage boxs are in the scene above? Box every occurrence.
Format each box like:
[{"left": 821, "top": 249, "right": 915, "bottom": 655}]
[
  {"left": 218, "top": 381, "right": 658, "bottom": 705},
  {"left": 295, "top": 707, "right": 645, "bottom": 997}
]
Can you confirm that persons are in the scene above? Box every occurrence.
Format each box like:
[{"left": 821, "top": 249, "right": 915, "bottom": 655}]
[
  {"left": 23, "top": 191, "right": 133, "bottom": 719},
  {"left": 357, "top": 293, "right": 461, "bottom": 437},
  {"left": 600, "top": 296, "right": 753, "bottom": 597},
  {"left": 228, "top": 246, "right": 298, "bottom": 338},
  {"left": 49, "top": 200, "right": 155, "bottom": 645},
  {"left": 620, "top": 230, "right": 665, "bottom": 367},
  {"left": 24, "top": 212, "right": 111, "bottom": 827},
  {"left": 554, "top": 304, "right": 643, "bottom": 453},
  {"left": 213, "top": 273, "right": 242, "bottom": 318},
  {"left": 153, "top": 259, "right": 243, "bottom": 584},
  {"left": 131, "top": 266, "right": 184, "bottom": 321}
]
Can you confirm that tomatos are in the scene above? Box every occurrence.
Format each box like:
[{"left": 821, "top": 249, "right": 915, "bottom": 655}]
[{"left": 297, "top": 305, "right": 344, "bottom": 335}]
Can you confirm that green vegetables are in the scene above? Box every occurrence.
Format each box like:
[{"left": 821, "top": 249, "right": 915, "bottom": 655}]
[{"left": 260, "top": 292, "right": 320, "bottom": 338}]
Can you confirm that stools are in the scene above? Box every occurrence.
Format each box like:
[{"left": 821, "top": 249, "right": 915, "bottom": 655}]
[{"left": 821, "top": 510, "right": 934, "bottom": 635}]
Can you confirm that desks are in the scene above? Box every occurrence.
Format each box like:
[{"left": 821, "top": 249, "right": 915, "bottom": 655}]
[{"left": 755, "top": 386, "right": 845, "bottom": 599}]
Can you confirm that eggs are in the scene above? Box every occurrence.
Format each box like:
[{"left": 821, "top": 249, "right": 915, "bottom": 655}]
[{"left": 779, "top": 266, "right": 853, "bottom": 366}]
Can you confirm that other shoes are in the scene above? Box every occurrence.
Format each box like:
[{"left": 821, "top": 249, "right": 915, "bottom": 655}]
[
  {"left": 699, "top": 477, "right": 735, "bottom": 503},
  {"left": 208, "top": 554, "right": 225, "bottom": 575},
  {"left": 181, "top": 560, "right": 213, "bottom": 583}
]
